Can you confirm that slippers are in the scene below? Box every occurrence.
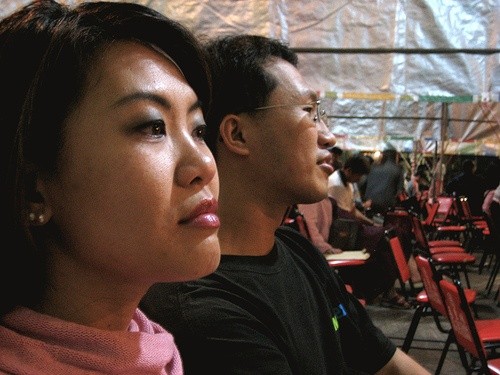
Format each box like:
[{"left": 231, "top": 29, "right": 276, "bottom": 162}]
[{"left": 381, "top": 293, "right": 411, "bottom": 309}]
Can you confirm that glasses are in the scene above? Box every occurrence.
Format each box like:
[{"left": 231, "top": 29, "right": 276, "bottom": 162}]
[{"left": 252, "top": 98, "right": 327, "bottom": 123}]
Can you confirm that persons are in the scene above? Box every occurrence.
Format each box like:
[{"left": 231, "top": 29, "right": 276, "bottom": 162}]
[
  {"left": 297, "top": 143, "right": 500, "bottom": 308},
  {"left": 1, "top": 0, "right": 223, "bottom": 375},
  {"left": 139, "top": 33, "right": 431, "bottom": 375}
]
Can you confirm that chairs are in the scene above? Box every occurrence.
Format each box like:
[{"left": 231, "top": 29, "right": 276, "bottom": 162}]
[{"left": 283, "top": 191, "right": 500, "bottom": 375}]
